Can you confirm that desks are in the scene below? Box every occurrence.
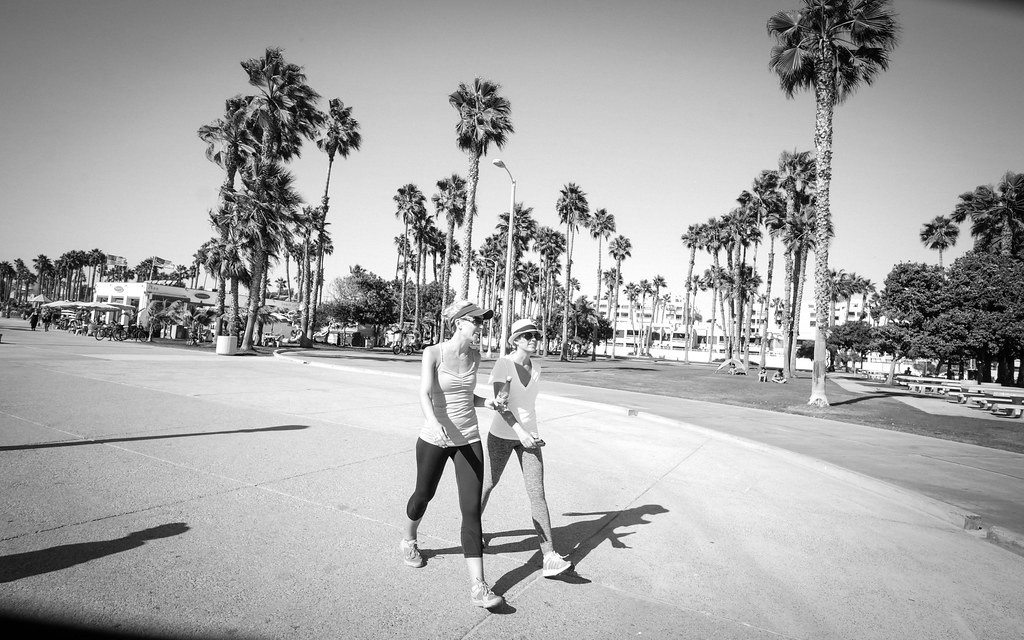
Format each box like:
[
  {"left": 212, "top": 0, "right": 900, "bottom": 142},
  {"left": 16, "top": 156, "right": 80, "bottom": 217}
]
[
  {"left": 983, "top": 389, "right": 1022, "bottom": 395},
  {"left": 992, "top": 391, "right": 1024, "bottom": 398},
  {"left": 906, "top": 377, "right": 948, "bottom": 382},
  {"left": 940, "top": 382, "right": 961, "bottom": 387},
  {"left": 965, "top": 384, "right": 1003, "bottom": 390},
  {"left": 896, "top": 376, "right": 920, "bottom": 380}
]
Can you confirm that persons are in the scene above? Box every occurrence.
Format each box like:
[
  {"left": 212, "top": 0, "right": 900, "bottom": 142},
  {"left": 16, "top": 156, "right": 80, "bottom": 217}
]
[
  {"left": 772, "top": 369, "right": 787, "bottom": 384},
  {"left": 42, "top": 311, "right": 51, "bottom": 332},
  {"left": 728, "top": 364, "right": 737, "bottom": 375},
  {"left": 28, "top": 309, "right": 41, "bottom": 331},
  {"left": 481, "top": 319, "right": 573, "bottom": 578},
  {"left": 400, "top": 299, "right": 507, "bottom": 609},
  {"left": 904, "top": 367, "right": 911, "bottom": 375},
  {"left": 759, "top": 367, "right": 767, "bottom": 382}
]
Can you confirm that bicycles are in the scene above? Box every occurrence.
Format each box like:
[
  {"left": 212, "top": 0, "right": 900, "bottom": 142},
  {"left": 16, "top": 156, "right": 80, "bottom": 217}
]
[
  {"left": 57, "top": 306, "right": 148, "bottom": 342},
  {"left": 391, "top": 328, "right": 441, "bottom": 356}
]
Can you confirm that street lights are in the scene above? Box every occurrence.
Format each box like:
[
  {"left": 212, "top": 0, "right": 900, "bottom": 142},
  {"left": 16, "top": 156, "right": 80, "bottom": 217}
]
[
  {"left": 492, "top": 158, "right": 515, "bottom": 353},
  {"left": 477, "top": 256, "right": 498, "bottom": 358}
]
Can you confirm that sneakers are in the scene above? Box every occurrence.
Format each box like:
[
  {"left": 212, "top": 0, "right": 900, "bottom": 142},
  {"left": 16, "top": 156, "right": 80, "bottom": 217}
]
[
  {"left": 469, "top": 577, "right": 503, "bottom": 609},
  {"left": 401, "top": 539, "right": 423, "bottom": 568},
  {"left": 542, "top": 550, "right": 574, "bottom": 578}
]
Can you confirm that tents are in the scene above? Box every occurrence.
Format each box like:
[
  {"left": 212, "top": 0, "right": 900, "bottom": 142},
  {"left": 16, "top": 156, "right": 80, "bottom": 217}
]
[
  {"left": 715, "top": 359, "right": 747, "bottom": 376},
  {"left": 39, "top": 300, "right": 136, "bottom": 313},
  {"left": 28, "top": 294, "right": 52, "bottom": 303}
]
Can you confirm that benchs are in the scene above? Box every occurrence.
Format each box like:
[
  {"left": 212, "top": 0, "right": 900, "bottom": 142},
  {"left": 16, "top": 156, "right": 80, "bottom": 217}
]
[{"left": 868, "top": 374, "right": 1024, "bottom": 416}]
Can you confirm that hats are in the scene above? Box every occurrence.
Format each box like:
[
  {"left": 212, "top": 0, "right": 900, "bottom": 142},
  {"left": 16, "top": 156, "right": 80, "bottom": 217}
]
[
  {"left": 507, "top": 319, "right": 542, "bottom": 346},
  {"left": 444, "top": 301, "right": 493, "bottom": 331}
]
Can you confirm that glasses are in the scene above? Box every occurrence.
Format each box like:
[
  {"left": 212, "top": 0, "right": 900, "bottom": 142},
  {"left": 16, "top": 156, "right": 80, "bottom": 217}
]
[
  {"left": 461, "top": 316, "right": 486, "bottom": 325},
  {"left": 522, "top": 332, "right": 542, "bottom": 340}
]
